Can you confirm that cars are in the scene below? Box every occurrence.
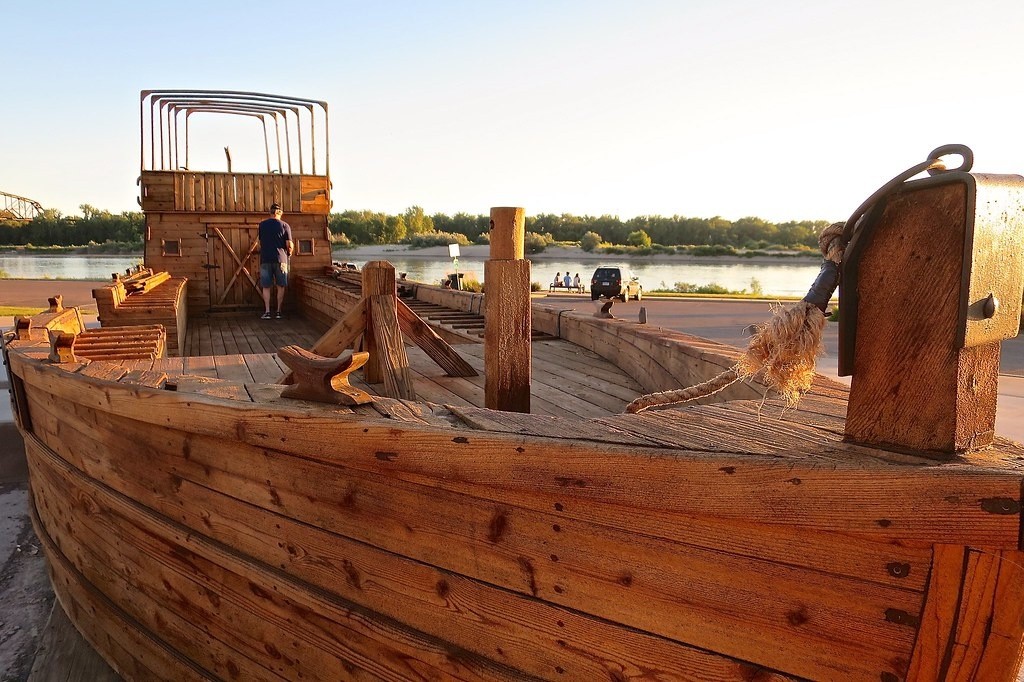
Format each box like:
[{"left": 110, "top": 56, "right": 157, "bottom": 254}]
[{"left": 590, "top": 266, "right": 643, "bottom": 302}]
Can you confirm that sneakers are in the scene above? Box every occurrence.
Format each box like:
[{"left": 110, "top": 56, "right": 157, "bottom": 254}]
[
  {"left": 276, "top": 312, "right": 282, "bottom": 318},
  {"left": 261, "top": 312, "right": 271, "bottom": 319}
]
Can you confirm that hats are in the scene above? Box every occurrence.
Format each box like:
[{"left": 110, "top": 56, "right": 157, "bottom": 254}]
[{"left": 271, "top": 204, "right": 280, "bottom": 209}]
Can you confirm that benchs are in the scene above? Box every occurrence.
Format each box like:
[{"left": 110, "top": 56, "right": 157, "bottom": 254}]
[{"left": 550, "top": 283, "right": 585, "bottom": 294}]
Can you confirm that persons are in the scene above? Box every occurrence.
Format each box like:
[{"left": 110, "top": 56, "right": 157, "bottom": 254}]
[
  {"left": 257, "top": 204, "right": 293, "bottom": 320},
  {"left": 554, "top": 272, "right": 561, "bottom": 287},
  {"left": 574, "top": 274, "right": 580, "bottom": 293},
  {"left": 564, "top": 272, "right": 572, "bottom": 293}
]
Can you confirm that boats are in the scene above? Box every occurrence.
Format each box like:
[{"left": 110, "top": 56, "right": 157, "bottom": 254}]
[{"left": 0, "top": 90, "right": 1024, "bottom": 682}]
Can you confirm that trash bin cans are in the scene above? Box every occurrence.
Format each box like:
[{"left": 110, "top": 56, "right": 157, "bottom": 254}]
[{"left": 446, "top": 273, "right": 465, "bottom": 291}]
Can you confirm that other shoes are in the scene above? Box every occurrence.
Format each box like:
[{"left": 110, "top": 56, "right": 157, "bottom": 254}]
[{"left": 560, "top": 284, "right": 562, "bottom": 286}]
